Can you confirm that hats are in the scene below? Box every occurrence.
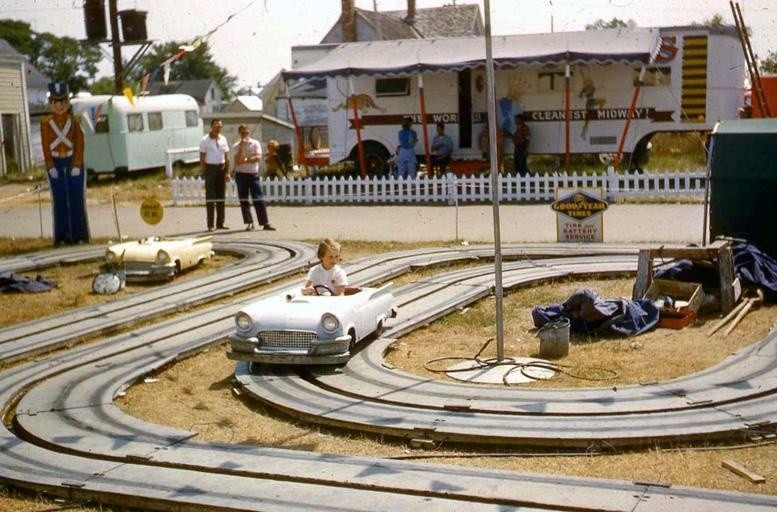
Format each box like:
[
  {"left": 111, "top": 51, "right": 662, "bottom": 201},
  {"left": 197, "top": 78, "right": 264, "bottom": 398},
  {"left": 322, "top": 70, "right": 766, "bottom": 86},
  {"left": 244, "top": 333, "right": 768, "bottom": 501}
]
[{"left": 46, "top": 81, "right": 72, "bottom": 101}]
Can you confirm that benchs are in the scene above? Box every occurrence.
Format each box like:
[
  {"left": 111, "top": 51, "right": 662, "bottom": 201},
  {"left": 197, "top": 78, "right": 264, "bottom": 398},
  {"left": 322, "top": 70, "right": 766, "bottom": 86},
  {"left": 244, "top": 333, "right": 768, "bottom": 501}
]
[{"left": 388, "top": 153, "right": 454, "bottom": 177}]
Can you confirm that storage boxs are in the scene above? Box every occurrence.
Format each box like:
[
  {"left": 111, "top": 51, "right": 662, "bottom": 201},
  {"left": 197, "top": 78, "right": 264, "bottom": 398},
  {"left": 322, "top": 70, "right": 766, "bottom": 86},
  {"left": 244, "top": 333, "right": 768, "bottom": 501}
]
[{"left": 641, "top": 277, "right": 705, "bottom": 319}]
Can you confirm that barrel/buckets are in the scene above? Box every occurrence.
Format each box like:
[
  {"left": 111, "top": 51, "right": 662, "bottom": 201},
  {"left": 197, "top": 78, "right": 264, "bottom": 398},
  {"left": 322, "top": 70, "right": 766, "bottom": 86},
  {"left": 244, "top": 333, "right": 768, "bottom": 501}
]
[{"left": 538, "top": 317, "right": 572, "bottom": 359}]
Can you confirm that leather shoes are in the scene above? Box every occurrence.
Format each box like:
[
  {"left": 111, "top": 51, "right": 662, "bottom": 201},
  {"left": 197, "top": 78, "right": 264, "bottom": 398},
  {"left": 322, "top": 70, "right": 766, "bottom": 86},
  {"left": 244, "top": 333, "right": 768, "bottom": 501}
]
[
  {"left": 246, "top": 223, "right": 255, "bottom": 232},
  {"left": 209, "top": 225, "right": 230, "bottom": 232},
  {"left": 263, "top": 223, "right": 277, "bottom": 232}
]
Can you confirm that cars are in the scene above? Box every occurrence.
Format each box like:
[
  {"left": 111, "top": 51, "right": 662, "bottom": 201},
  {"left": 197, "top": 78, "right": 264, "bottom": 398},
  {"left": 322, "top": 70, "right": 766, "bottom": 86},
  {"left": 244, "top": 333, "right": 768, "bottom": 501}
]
[{"left": 296, "top": 147, "right": 329, "bottom": 169}]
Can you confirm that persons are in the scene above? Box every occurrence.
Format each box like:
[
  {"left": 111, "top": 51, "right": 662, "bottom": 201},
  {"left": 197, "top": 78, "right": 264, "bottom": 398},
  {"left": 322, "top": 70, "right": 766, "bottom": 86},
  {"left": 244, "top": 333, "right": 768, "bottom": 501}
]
[
  {"left": 428, "top": 123, "right": 454, "bottom": 172},
  {"left": 396, "top": 117, "right": 418, "bottom": 179},
  {"left": 299, "top": 238, "right": 346, "bottom": 297},
  {"left": 197, "top": 120, "right": 231, "bottom": 231},
  {"left": 227, "top": 125, "right": 277, "bottom": 230},
  {"left": 386, "top": 145, "right": 418, "bottom": 179},
  {"left": 480, "top": 120, "right": 504, "bottom": 177},
  {"left": 511, "top": 114, "right": 531, "bottom": 180}
]
